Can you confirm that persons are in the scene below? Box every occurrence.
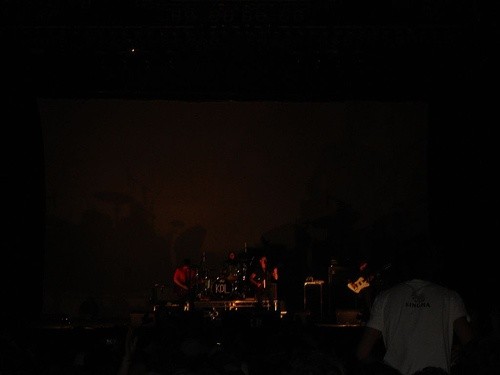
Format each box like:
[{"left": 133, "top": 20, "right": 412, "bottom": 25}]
[
  {"left": 0, "top": 251, "right": 499, "bottom": 374},
  {"left": 360, "top": 246, "right": 476, "bottom": 373}
]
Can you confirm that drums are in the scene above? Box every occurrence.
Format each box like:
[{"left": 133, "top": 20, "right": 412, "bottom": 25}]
[{"left": 212, "top": 279, "right": 232, "bottom": 298}]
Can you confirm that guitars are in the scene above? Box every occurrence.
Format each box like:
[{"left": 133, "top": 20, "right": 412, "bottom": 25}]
[{"left": 347, "top": 262, "right": 392, "bottom": 295}]
